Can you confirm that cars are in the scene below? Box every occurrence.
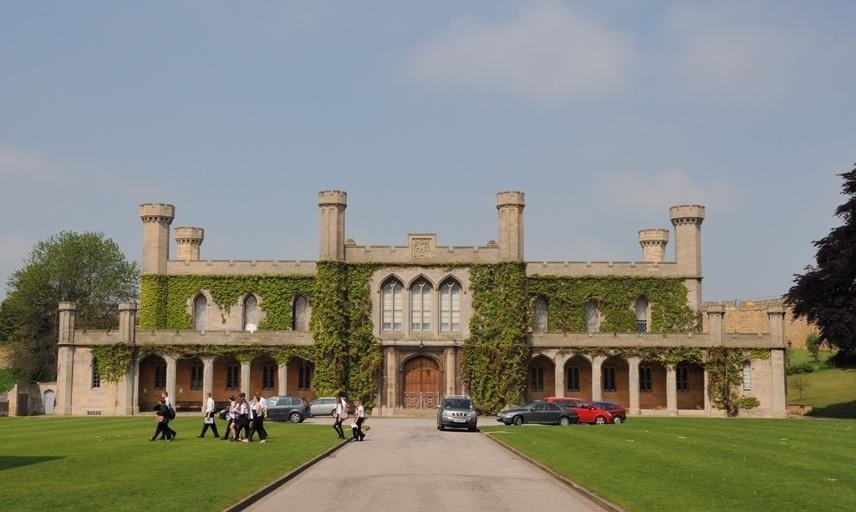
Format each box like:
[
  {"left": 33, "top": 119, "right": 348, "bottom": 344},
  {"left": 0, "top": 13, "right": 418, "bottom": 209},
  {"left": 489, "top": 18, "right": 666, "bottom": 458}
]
[
  {"left": 497, "top": 397, "right": 626, "bottom": 426},
  {"left": 219, "top": 396, "right": 356, "bottom": 423}
]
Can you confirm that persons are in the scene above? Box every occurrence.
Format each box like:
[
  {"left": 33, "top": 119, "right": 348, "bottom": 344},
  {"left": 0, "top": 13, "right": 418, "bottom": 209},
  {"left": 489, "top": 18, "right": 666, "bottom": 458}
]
[
  {"left": 332, "top": 396, "right": 366, "bottom": 440},
  {"left": 157, "top": 391, "right": 171, "bottom": 441},
  {"left": 197, "top": 392, "right": 219, "bottom": 438},
  {"left": 219, "top": 392, "right": 269, "bottom": 443},
  {"left": 147, "top": 397, "right": 177, "bottom": 440}
]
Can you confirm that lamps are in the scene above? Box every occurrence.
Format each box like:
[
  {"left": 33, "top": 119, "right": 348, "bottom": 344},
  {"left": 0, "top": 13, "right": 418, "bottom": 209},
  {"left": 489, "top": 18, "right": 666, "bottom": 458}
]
[
  {"left": 783, "top": 337, "right": 793, "bottom": 349},
  {"left": 417, "top": 340, "right": 426, "bottom": 350}
]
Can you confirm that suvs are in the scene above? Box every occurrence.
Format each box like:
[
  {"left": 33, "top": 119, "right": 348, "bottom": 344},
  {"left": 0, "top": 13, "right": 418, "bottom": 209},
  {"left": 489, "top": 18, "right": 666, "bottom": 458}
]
[{"left": 437, "top": 395, "right": 477, "bottom": 432}]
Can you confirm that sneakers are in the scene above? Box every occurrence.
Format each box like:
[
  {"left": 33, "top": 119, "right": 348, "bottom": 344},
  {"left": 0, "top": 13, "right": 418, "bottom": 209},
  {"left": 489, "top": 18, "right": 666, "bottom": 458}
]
[
  {"left": 148, "top": 431, "right": 176, "bottom": 442},
  {"left": 196, "top": 434, "right": 271, "bottom": 443},
  {"left": 338, "top": 434, "right": 366, "bottom": 441}
]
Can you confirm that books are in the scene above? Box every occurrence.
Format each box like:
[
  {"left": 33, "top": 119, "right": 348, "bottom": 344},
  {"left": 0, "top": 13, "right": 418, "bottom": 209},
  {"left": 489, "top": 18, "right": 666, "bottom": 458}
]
[{"left": 204, "top": 417, "right": 214, "bottom": 424}]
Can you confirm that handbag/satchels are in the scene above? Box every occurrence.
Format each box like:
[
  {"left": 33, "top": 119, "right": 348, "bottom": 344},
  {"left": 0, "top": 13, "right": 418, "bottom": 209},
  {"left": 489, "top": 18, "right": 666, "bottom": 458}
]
[
  {"left": 340, "top": 410, "right": 348, "bottom": 419},
  {"left": 168, "top": 407, "right": 176, "bottom": 420}
]
